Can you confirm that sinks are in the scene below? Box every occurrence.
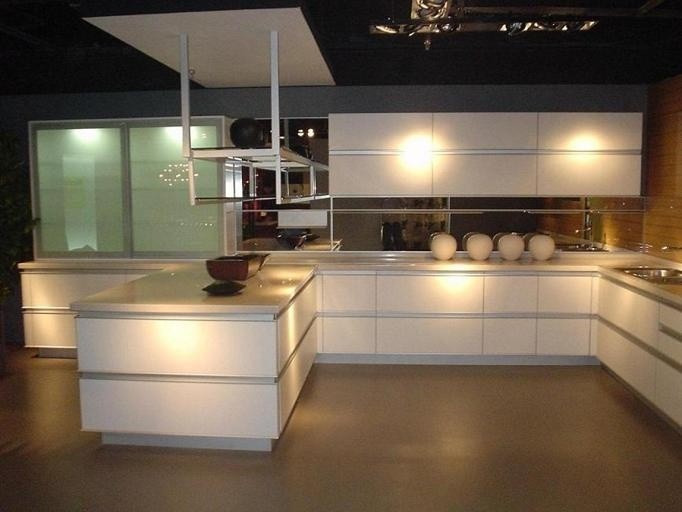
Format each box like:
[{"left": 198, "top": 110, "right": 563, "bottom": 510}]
[
  {"left": 563, "top": 249, "right": 601, "bottom": 251},
  {"left": 559, "top": 245, "right": 582, "bottom": 248},
  {"left": 645, "top": 278, "right": 682, "bottom": 284}
]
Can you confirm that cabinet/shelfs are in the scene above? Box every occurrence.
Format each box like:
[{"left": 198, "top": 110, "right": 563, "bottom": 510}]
[
  {"left": 315, "top": 274, "right": 600, "bottom": 373},
  {"left": 603, "top": 279, "right": 682, "bottom": 435},
  {"left": 29, "top": 115, "right": 242, "bottom": 261},
  {"left": 331, "top": 115, "right": 647, "bottom": 196}
]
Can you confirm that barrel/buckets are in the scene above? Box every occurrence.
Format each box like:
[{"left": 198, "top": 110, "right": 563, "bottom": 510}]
[{"left": 625, "top": 270, "right": 682, "bottom": 278}]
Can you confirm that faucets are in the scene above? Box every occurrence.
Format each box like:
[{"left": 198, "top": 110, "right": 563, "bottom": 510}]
[
  {"left": 576, "top": 227, "right": 598, "bottom": 250},
  {"left": 661, "top": 246, "right": 682, "bottom": 250}
]
